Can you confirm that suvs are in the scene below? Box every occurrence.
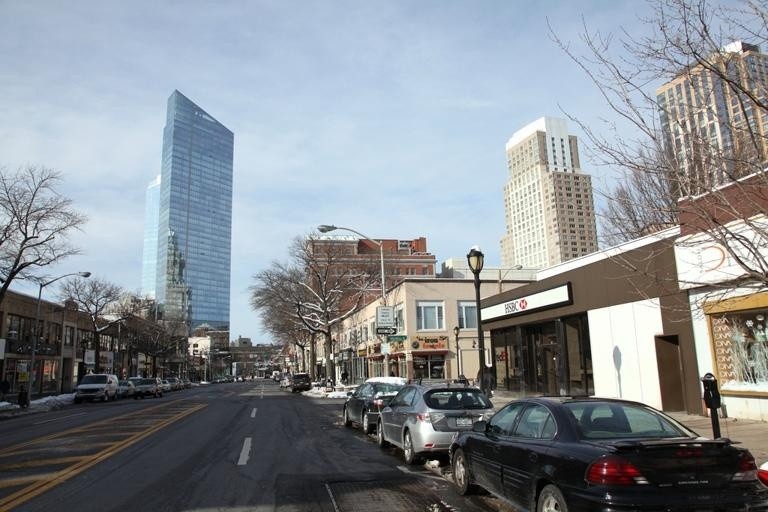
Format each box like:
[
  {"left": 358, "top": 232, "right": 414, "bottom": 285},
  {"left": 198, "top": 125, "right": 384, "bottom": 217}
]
[
  {"left": 265, "top": 370, "right": 312, "bottom": 395},
  {"left": 133, "top": 377, "right": 163, "bottom": 400}
]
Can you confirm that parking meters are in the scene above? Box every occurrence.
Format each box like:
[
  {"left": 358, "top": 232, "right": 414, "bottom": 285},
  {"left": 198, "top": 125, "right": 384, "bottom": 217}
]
[{"left": 700, "top": 372, "right": 721, "bottom": 439}]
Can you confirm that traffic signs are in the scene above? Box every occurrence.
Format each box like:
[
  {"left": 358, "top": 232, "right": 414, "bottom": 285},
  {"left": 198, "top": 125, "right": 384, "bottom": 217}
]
[
  {"left": 388, "top": 336, "right": 407, "bottom": 341},
  {"left": 377, "top": 328, "right": 397, "bottom": 335}
]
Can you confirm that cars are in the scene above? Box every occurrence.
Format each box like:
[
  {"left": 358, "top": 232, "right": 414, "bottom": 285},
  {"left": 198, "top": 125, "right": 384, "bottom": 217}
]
[
  {"left": 758, "top": 459, "right": 768, "bottom": 487},
  {"left": 167, "top": 376, "right": 191, "bottom": 392},
  {"left": 212, "top": 374, "right": 246, "bottom": 383},
  {"left": 448, "top": 394, "right": 767, "bottom": 512},
  {"left": 118, "top": 380, "right": 134, "bottom": 399},
  {"left": 161, "top": 380, "right": 170, "bottom": 392},
  {"left": 375, "top": 382, "right": 497, "bottom": 465},
  {"left": 343, "top": 377, "right": 400, "bottom": 434},
  {"left": 128, "top": 376, "right": 142, "bottom": 385}
]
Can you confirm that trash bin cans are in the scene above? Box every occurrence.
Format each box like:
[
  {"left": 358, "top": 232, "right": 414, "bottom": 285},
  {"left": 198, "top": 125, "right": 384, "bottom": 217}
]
[{"left": 18, "top": 392, "right": 27, "bottom": 407}]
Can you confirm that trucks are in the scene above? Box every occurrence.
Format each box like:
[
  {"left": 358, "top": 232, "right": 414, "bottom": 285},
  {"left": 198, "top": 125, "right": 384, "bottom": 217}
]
[{"left": 74, "top": 373, "right": 120, "bottom": 401}]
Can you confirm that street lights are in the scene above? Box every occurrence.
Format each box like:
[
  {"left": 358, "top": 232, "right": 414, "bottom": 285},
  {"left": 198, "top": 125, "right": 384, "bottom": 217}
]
[
  {"left": 204, "top": 349, "right": 219, "bottom": 382},
  {"left": 222, "top": 354, "right": 232, "bottom": 376},
  {"left": 453, "top": 326, "right": 460, "bottom": 380},
  {"left": 466, "top": 248, "right": 494, "bottom": 409},
  {"left": 317, "top": 224, "right": 390, "bottom": 378},
  {"left": 497, "top": 264, "right": 524, "bottom": 294},
  {"left": 25, "top": 270, "right": 92, "bottom": 407}
]
